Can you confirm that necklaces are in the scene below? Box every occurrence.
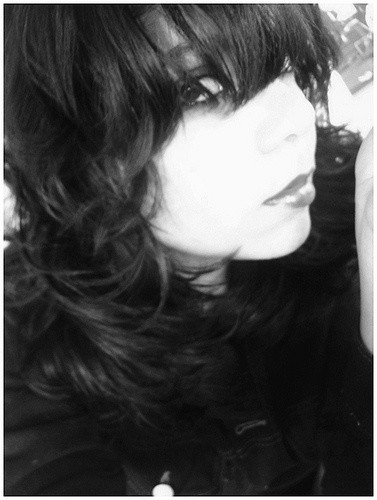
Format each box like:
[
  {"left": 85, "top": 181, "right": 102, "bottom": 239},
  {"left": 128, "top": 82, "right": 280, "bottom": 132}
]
[{"left": 3, "top": 5, "right": 370, "bottom": 497}]
